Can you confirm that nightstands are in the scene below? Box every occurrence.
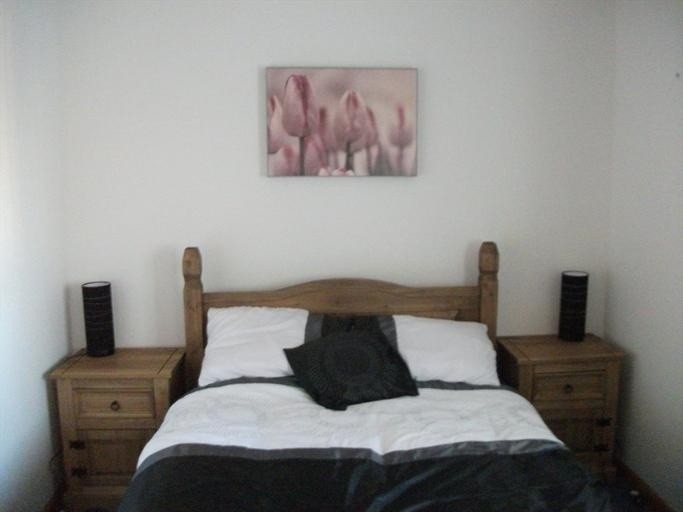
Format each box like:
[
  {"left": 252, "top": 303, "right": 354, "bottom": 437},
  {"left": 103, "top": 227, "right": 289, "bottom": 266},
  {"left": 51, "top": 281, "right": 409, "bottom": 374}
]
[
  {"left": 48, "top": 346, "right": 186, "bottom": 512},
  {"left": 496, "top": 334, "right": 624, "bottom": 460}
]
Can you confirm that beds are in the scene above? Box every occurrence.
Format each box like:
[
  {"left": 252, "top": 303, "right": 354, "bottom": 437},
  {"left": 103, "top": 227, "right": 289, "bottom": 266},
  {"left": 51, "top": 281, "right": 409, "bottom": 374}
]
[{"left": 115, "top": 240, "right": 618, "bottom": 512}]
